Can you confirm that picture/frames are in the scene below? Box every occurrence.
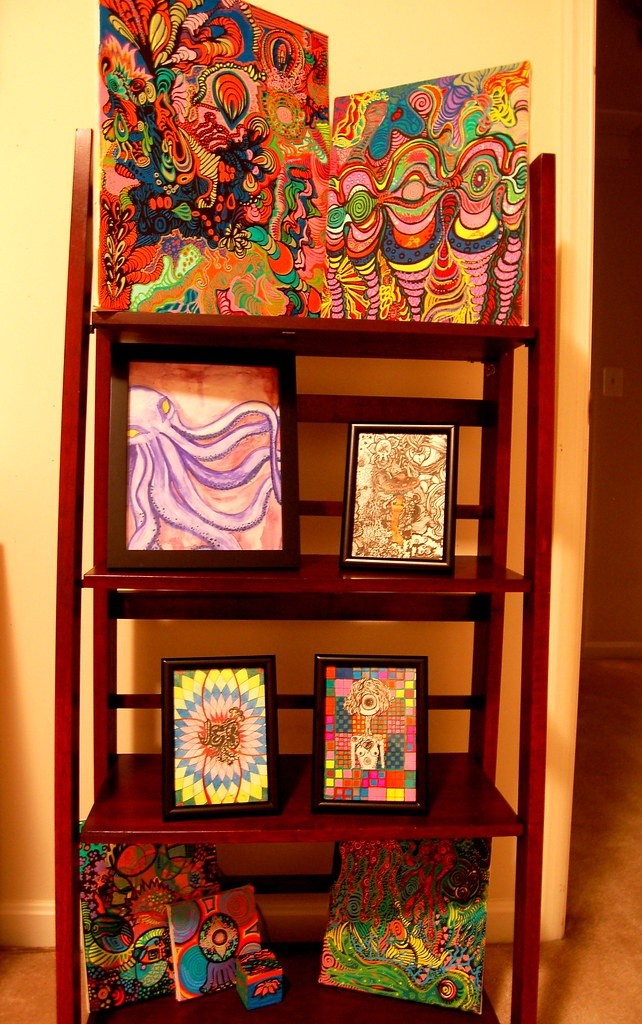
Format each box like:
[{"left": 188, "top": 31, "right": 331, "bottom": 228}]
[
  {"left": 158, "top": 655, "right": 278, "bottom": 819},
  {"left": 311, "top": 653, "right": 430, "bottom": 816},
  {"left": 339, "top": 418, "right": 459, "bottom": 573},
  {"left": 107, "top": 340, "right": 301, "bottom": 573}
]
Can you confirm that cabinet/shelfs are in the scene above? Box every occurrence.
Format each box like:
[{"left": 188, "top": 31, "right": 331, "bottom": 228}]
[{"left": 53, "top": 127, "right": 559, "bottom": 1024}]
